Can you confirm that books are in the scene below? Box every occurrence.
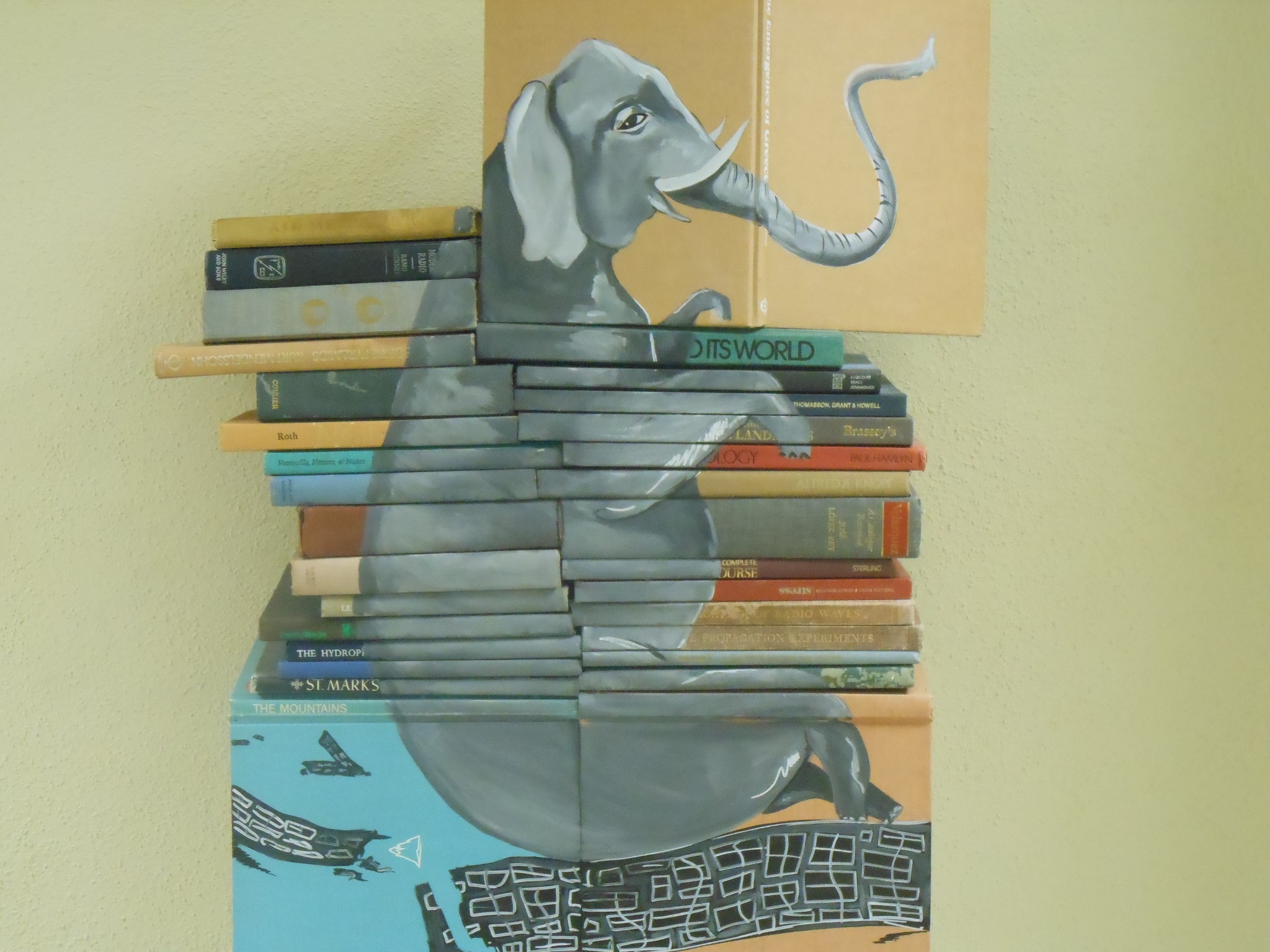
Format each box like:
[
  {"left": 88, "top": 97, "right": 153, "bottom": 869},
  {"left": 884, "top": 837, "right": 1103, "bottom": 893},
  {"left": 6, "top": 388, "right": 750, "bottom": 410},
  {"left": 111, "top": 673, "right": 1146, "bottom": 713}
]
[
  {"left": 155, "top": 204, "right": 927, "bottom": 695},
  {"left": 482, "top": 0, "right": 987, "bottom": 337}
]
